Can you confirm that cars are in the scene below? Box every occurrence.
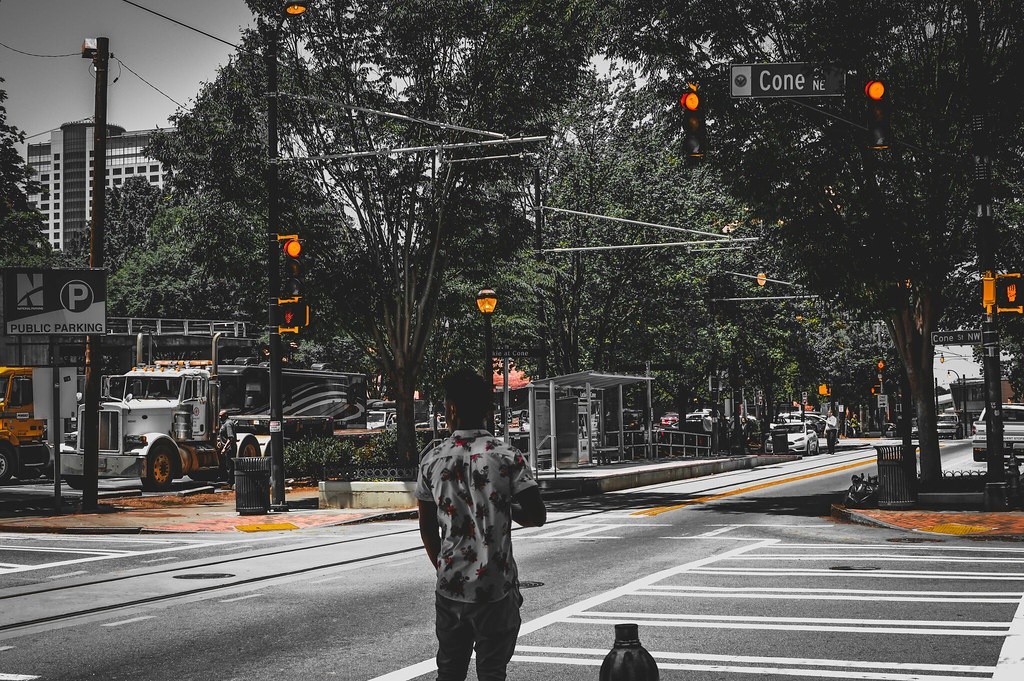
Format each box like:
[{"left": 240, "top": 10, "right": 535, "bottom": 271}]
[
  {"left": 770, "top": 410, "right": 851, "bottom": 438},
  {"left": 765, "top": 423, "right": 820, "bottom": 458},
  {"left": 0, "top": 366, "right": 54, "bottom": 487},
  {"left": 885, "top": 421, "right": 920, "bottom": 439},
  {"left": 367, "top": 410, "right": 447, "bottom": 432},
  {"left": 654, "top": 407, "right": 762, "bottom": 448}
]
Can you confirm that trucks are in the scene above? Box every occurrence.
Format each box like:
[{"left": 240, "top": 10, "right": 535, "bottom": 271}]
[{"left": 935, "top": 413, "right": 959, "bottom": 439}]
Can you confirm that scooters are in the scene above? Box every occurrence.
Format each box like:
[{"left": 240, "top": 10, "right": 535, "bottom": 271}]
[{"left": 845, "top": 472, "right": 879, "bottom": 510}]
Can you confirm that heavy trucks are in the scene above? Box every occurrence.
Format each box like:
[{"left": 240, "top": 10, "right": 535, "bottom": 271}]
[{"left": 57, "top": 322, "right": 369, "bottom": 494}]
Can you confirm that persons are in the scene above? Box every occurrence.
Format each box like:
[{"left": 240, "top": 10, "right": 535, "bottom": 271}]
[
  {"left": 851, "top": 414, "right": 862, "bottom": 438},
  {"left": 217, "top": 410, "right": 237, "bottom": 490},
  {"left": 824, "top": 410, "right": 837, "bottom": 455},
  {"left": 741, "top": 417, "right": 749, "bottom": 455},
  {"left": 414, "top": 368, "right": 546, "bottom": 681}
]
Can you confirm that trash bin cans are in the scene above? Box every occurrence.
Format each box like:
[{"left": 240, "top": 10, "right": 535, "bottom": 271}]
[
  {"left": 231, "top": 456, "right": 274, "bottom": 516},
  {"left": 872, "top": 445, "right": 920, "bottom": 510},
  {"left": 772, "top": 429, "right": 788, "bottom": 455}
]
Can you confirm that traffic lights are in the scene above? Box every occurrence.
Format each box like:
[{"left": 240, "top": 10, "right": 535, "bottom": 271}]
[
  {"left": 278, "top": 300, "right": 309, "bottom": 328},
  {"left": 680, "top": 87, "right": 706, "bottom": 164},
  {"left": 995, "top": 274, "right": 1024, "bottom": 308},
  {"left": 285, "top": 238, "right": 305, "bottom": 296},
  {"left": 865, "top": 80, "right": 891, "bottom": 150}
]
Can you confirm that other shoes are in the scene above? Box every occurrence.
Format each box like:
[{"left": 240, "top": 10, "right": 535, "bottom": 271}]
[
  {"left": 220, "top": 485, "right": 232, "bottom": 490},
  {"left": 826, "top": 452, "right": 835, "bottom": 455}
]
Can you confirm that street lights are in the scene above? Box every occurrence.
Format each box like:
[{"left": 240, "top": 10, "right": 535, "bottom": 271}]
[
  {"left": 263, "top": 0, "right": 308, "bottom": 513},
  {"left": 476, "top": 288, "right": 497, "bottom": 438},
  {"left": 947, "top": 369, "right": 960, "bottom": 422}
]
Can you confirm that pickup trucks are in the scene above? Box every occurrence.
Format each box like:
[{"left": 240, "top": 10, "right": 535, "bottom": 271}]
[{"left": 972, "top": 403, "right": 1024, "bottom": 463}]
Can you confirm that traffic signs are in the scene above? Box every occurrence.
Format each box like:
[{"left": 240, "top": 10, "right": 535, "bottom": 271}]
[{"left": 878, "top": 395, "right": 888, "bottom": 407}]
